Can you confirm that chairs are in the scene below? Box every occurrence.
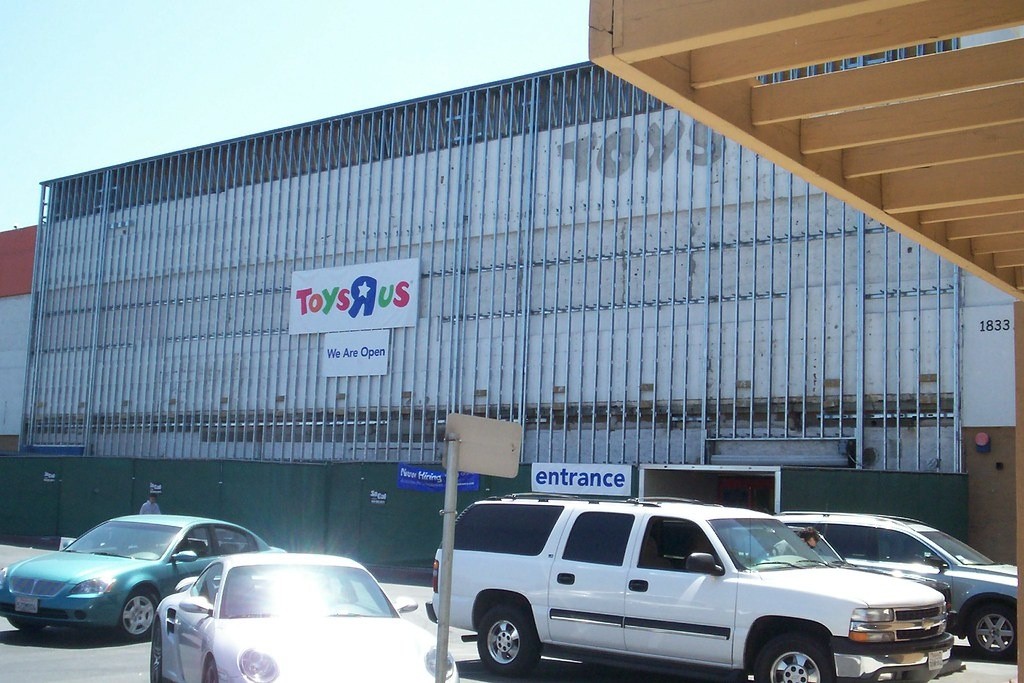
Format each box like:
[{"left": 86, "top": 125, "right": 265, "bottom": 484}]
[
  {"left": 179, "top": 540, "right": 208, "bottom": 557},
  {"left": 219, "top": 543, "right": 241, "bottom": 554}
]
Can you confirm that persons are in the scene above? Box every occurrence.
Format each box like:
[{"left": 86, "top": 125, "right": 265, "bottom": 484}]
[
  {"left": 139, "top": 494, "right": 161, "bottom": 515},
  {"left": 766, "top": 526, "right": 820, "bottom": 556}
]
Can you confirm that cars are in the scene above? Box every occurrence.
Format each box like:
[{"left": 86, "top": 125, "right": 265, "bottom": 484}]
[{"left": 0, "top": 512, "right": 289, "bottom": 643}]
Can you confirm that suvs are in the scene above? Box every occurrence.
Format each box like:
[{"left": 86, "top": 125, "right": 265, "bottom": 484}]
[
  {"left": 761, "top": 508, "right": 1018, "bottom": 662},
  {"left": 425, "top": 489, "right": 955, "bottom": 682}
]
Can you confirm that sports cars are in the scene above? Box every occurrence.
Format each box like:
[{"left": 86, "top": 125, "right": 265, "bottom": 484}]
[{"left": 150, "top": 552, "right": 460, "bottom": 683}]
